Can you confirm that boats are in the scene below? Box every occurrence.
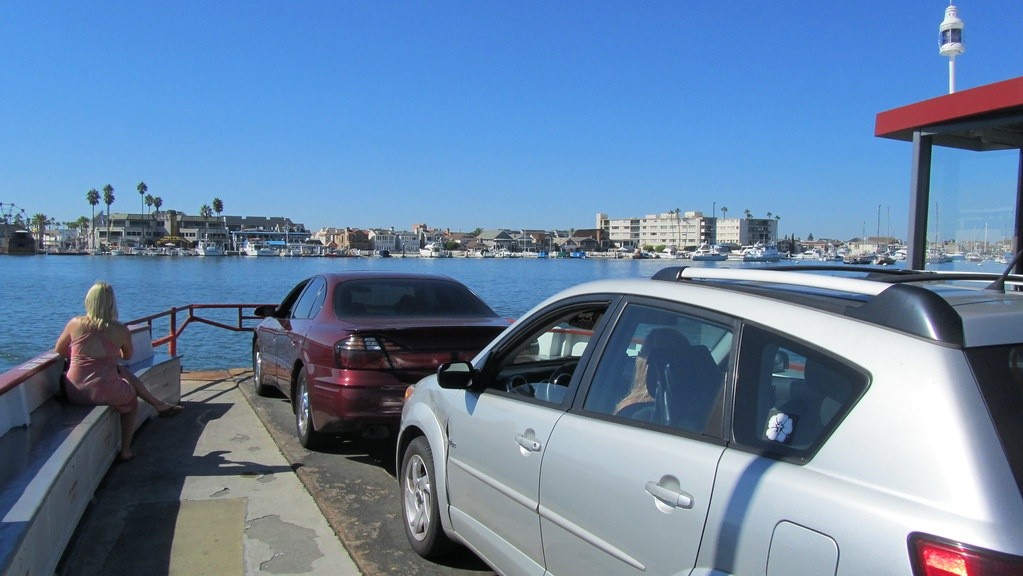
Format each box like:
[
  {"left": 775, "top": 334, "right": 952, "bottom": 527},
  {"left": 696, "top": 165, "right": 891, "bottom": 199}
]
[
  {"left": 690, "top": 250, "right": 728, "bottom": 261},
  {"left": 819, "top": 253, "right": 897, "bottom": 265}
]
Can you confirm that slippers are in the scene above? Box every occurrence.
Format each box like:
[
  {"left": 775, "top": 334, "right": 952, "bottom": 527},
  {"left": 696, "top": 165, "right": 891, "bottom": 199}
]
[
  {"left": 120, "top": 451, "right": 136, "bottom": 461},
  {"left": 157, "top": 405, "right": 185, "bottom": 417}
]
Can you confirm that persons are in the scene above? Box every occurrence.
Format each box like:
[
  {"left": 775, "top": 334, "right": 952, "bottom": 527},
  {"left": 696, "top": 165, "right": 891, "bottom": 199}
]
[
  {"left": 613, "top": 327, "right": 689, "bottom": 419},
  {"left": 55, "top": 282, "right": 183, "bottom": 460}
]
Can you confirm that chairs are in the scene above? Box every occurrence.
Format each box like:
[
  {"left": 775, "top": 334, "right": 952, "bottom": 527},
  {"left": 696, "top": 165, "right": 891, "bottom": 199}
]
[
  {"left": 394, "top": 294, "right": 413, "bottom": 308},
  {"left": 615, "top": 345, "right": 718, "bottom": 434}
]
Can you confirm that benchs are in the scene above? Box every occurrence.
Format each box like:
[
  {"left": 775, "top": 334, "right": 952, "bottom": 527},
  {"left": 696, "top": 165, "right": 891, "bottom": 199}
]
[{"left": 0, "top": 325, "right": 185, "bottom": 576}]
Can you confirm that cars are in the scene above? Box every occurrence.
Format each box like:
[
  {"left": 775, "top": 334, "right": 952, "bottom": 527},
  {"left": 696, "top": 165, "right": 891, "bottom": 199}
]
[
  {"left": 395, "top": 266, "right": 1023, "bottom": 575},
  {"left": 251, "top": 272, "right": 517, "bottom": 452}
]
